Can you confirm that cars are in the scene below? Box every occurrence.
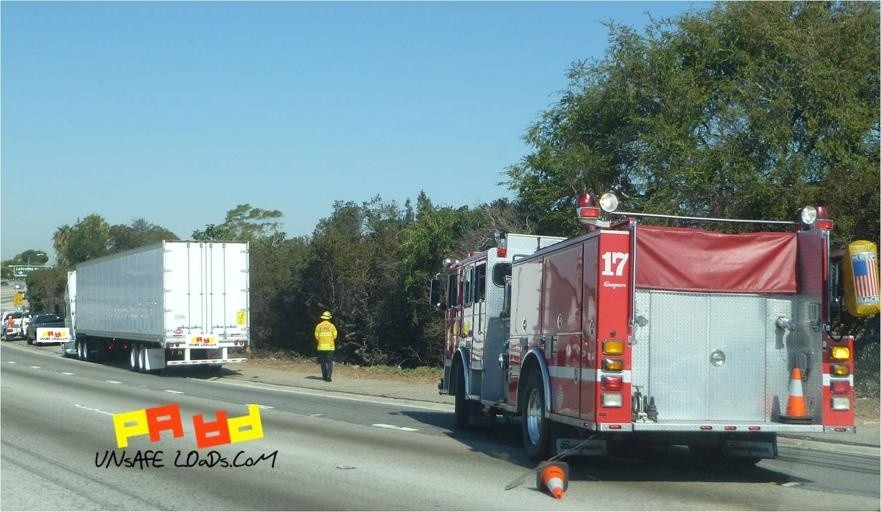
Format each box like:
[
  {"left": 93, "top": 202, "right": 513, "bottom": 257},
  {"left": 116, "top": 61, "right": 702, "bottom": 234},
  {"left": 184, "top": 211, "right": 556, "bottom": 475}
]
[{"left": 0, "top": 311, "right": 48, "bottom": 340}]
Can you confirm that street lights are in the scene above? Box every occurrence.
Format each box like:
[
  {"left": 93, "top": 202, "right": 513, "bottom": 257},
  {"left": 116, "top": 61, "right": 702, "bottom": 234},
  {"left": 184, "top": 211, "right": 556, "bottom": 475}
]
[{"left": 18, "top": 253, "right": 45, "bottom": 273}]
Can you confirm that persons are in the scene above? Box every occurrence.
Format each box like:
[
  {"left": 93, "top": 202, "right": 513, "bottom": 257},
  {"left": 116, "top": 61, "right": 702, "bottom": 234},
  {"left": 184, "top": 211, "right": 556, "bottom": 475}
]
[{"left": 314, "top": 310, "right": 337, "bottom": 381}]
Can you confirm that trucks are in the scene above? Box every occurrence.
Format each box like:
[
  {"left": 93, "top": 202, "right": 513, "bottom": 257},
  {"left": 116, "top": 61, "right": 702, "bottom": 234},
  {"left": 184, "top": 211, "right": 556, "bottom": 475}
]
[{"left": 54, "top": 240, "right": 252, "bottom": 374}]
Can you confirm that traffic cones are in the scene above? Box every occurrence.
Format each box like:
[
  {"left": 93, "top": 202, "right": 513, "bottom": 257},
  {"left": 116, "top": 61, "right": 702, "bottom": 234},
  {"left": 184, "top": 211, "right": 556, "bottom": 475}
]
[
  {"left": 778, "top": 366, "right": 814, "bottom": 425},
  {"left": 537, "top": 461, "right": 569, "bottom": 498}
]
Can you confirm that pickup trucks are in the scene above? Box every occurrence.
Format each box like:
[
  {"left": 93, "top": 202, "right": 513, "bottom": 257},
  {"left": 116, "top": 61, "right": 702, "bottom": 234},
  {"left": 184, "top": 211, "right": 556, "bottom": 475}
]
[{"left": 26, "top": 313, "right": 65, "bottom": 346}]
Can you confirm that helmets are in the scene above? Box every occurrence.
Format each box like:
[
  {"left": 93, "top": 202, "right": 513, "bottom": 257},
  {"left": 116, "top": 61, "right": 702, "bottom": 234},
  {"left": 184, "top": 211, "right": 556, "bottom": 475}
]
[{"left": 320, "top": 311, "right": 332, "bottom": 319}]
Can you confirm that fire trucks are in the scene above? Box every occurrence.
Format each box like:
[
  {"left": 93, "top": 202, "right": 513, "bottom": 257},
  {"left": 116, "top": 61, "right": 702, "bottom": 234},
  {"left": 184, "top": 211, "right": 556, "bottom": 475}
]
[{"left": 431, "top": 193, "right": 857, "bottom": 472}]
